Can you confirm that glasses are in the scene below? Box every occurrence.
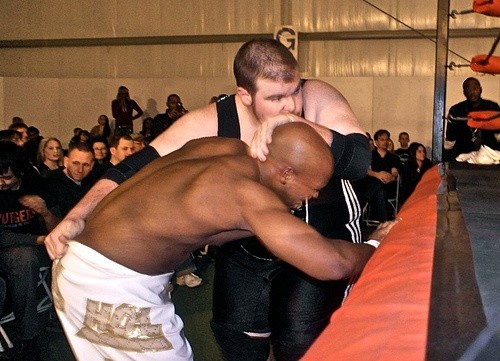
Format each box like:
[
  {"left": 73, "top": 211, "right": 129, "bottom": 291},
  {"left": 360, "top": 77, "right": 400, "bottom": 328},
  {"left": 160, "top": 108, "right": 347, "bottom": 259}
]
[{"left": 134, "top": 140, "right": 144, "bottom": 144}]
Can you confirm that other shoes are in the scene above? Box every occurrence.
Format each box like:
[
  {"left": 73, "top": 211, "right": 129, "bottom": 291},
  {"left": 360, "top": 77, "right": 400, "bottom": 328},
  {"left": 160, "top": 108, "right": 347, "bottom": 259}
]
[
  {"left": 48, "top": 314, "right": 59, "bottom": 324},
  {"left": 17, "top": 336, "right": 41, "bottom": 360}
]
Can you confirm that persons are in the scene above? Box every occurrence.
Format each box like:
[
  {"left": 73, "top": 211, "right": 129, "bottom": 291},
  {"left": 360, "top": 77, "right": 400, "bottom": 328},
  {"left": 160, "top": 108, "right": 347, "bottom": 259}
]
[
  {"left": 42, "top": 37, "right": 372, "bottom": 361},
  {"left": 0, "top": 86, "right": 227, "bottom": 361},
  {"left": 445, "top": 76, "right": 500, "bottom": 169},
  {"left": 52, "top": 121, "right": 403, "bottom": 361},
  {"left": 365, "top": 129, "right": 427, "bottom": 184}
]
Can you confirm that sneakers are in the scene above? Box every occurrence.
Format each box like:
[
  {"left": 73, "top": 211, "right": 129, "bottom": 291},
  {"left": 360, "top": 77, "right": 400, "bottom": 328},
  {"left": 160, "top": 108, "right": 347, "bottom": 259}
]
[{"left": 175, "top": 272, "right": 202, "bottom": 288}]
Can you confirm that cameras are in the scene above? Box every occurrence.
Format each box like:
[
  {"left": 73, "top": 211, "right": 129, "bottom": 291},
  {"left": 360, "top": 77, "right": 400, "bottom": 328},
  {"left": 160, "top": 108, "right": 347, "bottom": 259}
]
[{"left": 176, "top": 102, "right": 182, "bottom": 111}]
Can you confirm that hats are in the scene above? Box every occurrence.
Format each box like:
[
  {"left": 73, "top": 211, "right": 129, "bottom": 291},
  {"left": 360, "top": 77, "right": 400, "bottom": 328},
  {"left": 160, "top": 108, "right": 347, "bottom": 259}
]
[{"left": 130, "top": 134, "right": 144, "bottom": 142}]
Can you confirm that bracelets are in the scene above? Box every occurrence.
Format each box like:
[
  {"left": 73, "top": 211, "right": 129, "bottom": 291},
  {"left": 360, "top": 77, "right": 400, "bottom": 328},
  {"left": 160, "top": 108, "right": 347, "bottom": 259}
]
[{"left": 363, "top": 239, "right": 379, "bottom": 249}]
